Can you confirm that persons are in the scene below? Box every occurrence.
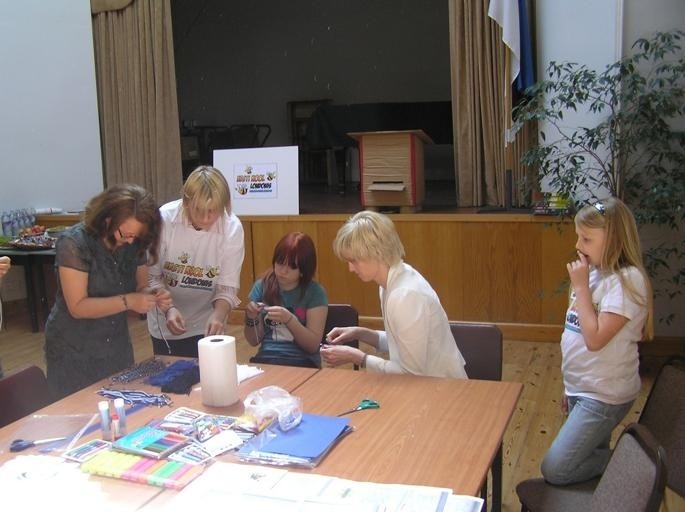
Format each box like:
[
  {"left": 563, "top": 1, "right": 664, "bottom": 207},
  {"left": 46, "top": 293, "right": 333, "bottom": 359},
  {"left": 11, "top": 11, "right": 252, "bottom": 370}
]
[
  {"left": 320, "top": 210, "right": 469, "bottom": 379},
  {"left": 43, "top": 183, "right": 173, "bottom": 403},
  {"left": 0, "top": 255, "right": 11, "bottom": 281},
  {"left": 244, "top": 232, "right": 329, "bottom": 369},
  {"left": 540, "top": 196, "right": 655, "bottom": 487},
  {"left": 147, "top": 165, "right": 245, "bottom": 357}
]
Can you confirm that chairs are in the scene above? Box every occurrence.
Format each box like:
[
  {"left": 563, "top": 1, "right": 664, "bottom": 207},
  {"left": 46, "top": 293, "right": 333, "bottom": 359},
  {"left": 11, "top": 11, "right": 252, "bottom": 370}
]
[
  {"left": 180, "top": 135, "right": 202, "bottom": 167},
  {"left": 0, "top": 365, "right": 50, "bottom": 427},
  {"left": 584, "top": 423, "right": 669, "bottom": 512},
  {"left": 515, "top": 353, "right": 685, "bottom": 511},
  {"left": 449, "top": 322, "right": 503, "bottom": 512},
  {"left": 321, "top": 304, "right": 360, "bottom": 370},
  {"left": 286, "top": 98, "right": 346, "bottom": 186}
]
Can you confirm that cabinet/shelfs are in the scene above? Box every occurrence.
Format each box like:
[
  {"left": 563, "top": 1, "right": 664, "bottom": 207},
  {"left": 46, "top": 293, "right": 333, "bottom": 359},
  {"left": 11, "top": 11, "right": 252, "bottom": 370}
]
[{"left": 30, "top": 205, "right": 579, "bottom": 342}]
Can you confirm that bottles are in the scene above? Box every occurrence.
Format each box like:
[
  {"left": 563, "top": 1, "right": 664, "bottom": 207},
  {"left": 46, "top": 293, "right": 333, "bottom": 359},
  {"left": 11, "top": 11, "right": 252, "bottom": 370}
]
[{"left": 2, "top": 208, "right": 37, "bottom": 238}]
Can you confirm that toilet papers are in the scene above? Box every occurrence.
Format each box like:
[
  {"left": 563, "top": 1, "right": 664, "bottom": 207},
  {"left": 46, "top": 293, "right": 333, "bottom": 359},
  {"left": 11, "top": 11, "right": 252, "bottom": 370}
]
[{"left": 198, "top": 335, "right": 239, "bottom": 407}]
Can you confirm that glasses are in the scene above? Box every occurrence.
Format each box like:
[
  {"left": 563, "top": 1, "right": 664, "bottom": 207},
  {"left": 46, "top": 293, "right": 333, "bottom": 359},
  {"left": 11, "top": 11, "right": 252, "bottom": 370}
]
[{"left": 116, "top": 226, "right": 139, "bottom": 240}]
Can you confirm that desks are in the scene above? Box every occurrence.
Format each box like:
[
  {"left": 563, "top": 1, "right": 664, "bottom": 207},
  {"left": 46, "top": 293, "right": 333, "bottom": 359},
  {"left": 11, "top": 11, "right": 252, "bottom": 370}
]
[
  {"left": 138, "top": 367, "right": 526, "bottom": 512},
  {"left": 0, "top": 351, "right": 320, "bottom": 512},
  {"left": 0, "top": 246, "right": 56, "bottom": 333},
  {"left": 302, "top": 101, "right": 453, "bottom": 199}
]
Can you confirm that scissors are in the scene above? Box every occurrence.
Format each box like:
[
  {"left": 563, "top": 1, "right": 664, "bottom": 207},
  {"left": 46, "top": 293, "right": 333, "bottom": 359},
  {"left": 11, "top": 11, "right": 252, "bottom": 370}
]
[
  {"left": 336, "top": 399, "right": 380, "bottom": 418},
  {"left": 9, "top": 437, "right": 67, "bottom": 453}
]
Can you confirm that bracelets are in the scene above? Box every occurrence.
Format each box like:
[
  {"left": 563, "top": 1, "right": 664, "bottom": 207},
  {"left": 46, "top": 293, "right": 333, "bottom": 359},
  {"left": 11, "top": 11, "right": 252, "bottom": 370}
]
[
  {"left": 284, "top": 313, "right": 294, "bottom": 325},
  {"left": 119, "top": 294, "right": 130, "bottom": 311},
  {"left": 164, "top": 305, "right": 175, "bottom": 317},
  {"left": 246, "top": 318, "right": 259, "bottom": 327},
  {"left": 360, "top": 353, "right": 367, "bottom": 368}
]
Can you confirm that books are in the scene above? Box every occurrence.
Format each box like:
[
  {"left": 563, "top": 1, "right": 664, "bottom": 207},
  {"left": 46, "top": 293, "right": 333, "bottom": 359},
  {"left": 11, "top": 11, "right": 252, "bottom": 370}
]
[{"left": 532, "top": 189, "right": 577, "bottom": 216}]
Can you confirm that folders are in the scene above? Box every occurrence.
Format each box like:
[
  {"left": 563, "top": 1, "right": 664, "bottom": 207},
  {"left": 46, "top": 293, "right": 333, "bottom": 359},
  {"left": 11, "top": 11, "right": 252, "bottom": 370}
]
[{"left": 237, "top": 414, "right": 350, "bottom": 467}]
